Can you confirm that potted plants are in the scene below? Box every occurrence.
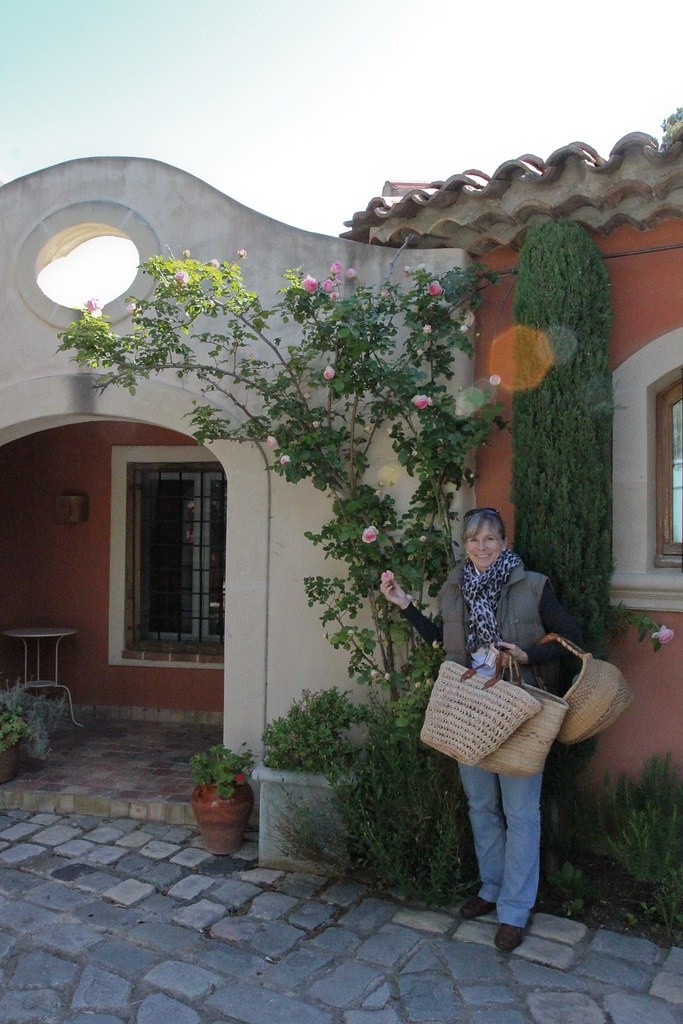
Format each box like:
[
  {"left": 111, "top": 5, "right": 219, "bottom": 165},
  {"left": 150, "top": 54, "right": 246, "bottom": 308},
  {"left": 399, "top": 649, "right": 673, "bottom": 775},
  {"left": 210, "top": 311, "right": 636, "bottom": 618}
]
[
  {"left": 251, "top": 687, "right": 377, "bottom": 868},
  {"left": 0, "top": 677, "right": 69, "bottom": 784}
]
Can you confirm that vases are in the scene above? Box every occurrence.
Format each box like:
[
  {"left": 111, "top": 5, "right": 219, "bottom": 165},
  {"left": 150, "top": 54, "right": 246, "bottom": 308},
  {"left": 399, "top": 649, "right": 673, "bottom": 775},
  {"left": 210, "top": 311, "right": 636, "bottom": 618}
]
[{"left": 191, "top": 779, "right": 254, "bottom": 854}]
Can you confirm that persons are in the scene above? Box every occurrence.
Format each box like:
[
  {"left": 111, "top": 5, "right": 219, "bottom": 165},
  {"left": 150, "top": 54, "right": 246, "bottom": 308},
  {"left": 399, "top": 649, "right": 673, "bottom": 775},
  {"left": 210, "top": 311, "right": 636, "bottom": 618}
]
[{"left": 380, "top": 507, "right": 584, "bottom": 952}]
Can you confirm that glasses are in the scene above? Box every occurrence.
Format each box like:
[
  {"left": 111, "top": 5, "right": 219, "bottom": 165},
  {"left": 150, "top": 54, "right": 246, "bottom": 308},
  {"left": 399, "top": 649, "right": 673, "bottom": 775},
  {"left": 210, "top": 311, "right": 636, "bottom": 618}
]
[{"left": 464, "top": 507, "right": 500, "bottom": 521}]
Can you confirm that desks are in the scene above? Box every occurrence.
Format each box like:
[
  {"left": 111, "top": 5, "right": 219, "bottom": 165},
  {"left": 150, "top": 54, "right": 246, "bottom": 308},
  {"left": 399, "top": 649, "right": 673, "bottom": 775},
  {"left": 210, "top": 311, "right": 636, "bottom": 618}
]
[{"left": 0, "top": 628, "right": 84, "bottom": 728}]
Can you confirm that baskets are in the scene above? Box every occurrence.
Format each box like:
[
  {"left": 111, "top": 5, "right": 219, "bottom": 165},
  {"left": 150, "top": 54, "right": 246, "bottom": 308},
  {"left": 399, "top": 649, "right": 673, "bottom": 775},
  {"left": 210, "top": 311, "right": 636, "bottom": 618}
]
[{"left": 420, "top": 633, "right": 634, "bottom": 776}]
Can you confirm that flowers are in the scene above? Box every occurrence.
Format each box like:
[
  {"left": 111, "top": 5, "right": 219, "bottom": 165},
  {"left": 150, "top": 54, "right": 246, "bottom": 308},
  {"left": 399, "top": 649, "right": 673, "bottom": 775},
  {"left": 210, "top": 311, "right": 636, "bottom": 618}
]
[{"left": 187, "top": 742, "right": 258, "bottom": 799}]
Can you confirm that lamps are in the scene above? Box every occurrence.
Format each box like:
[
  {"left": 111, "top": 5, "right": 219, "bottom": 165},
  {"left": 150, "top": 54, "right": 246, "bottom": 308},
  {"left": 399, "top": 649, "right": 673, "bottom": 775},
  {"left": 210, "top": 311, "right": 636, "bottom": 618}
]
[{"left": 53, "top": 492, "right": 88, "bottom": 525}]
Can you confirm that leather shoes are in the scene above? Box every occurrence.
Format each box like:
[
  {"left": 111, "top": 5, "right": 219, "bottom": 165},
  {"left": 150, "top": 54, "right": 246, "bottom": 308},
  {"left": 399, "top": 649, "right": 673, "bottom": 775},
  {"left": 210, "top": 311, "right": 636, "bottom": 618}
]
[
  {"left": 496, "top": 924, "right": 524, "bottom": 950},
  {"left": 459, "top": 895, "right": 496, "bottom": 919}
]
